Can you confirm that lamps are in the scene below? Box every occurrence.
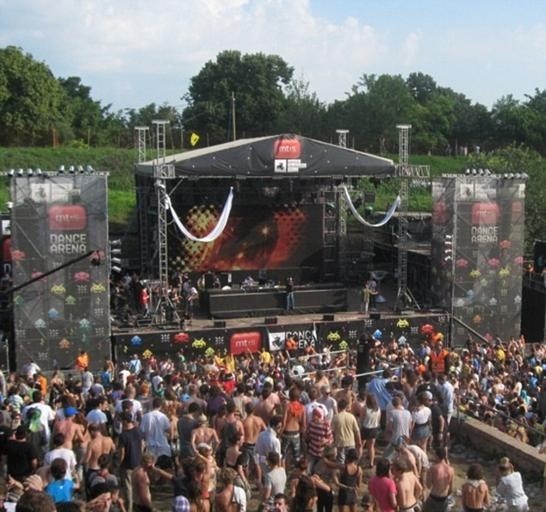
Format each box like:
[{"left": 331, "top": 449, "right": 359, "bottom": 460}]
[
  {"left": 108, "top": 238, "right": 122, "bottom": 273},
  {"left": 464, "top": 168, "right": 530, "bottom": 180},
  {"left": 7, "top": 163, "right": 94, "bottom": 177},
  {"left": 442, "top": 234, "right": 454, "bottom": 263}
]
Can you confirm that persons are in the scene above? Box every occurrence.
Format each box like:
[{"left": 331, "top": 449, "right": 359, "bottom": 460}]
[
  {"left": 0, "top": 333, "right": 546, "bottom": 512},
  {"left": 117, "top": 264, "right": 222, "bottom": 327},
  {"left": 357, "top": 284, "right": 378, "bottom": 317},
  {"left": 243, "top": 275, "right": 254, "bottom": 286},
  {"left": 285, "top": 275, "right": 296, "bottom": 311},
  {"left": 365, "top": 277, "right": 377, "bottom": 308}
]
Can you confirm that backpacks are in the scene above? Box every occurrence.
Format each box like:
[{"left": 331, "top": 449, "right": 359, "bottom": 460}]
[{"left": 298, "top": 473, "right": 315, "bottom": 504}]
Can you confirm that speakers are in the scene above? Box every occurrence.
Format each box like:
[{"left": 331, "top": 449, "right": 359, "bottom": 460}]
[
  {"left": 324, "top": 315, "right": 333, "bottom": 321},
  {"left": 370, "top": 314, "right": 380, "bottom": 319},
  {"left": 214, "top": 322, "right": 225, "bottom": 327},
  {"left": 265, "top": 317, "right": 277, "bottom": 324}
]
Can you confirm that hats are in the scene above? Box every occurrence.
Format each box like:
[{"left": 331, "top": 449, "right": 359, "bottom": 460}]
[
  {"left": 198, "top": 415, "right": 208, "bottom": 422},
  {"left": 172, "top": 495, "right": 190, "bottom": 512},
  {"left": 420, "top": 391, "right": 433, "bottom": 400},
  {"left": 391, "top": 435, "right": 405, "bottom": 446},
  {"left": 65, "top": 406, "right": 80, "bottom": 416},
  {"left": 27, "top": 408, "right": 41, "bottom": 420}
]
